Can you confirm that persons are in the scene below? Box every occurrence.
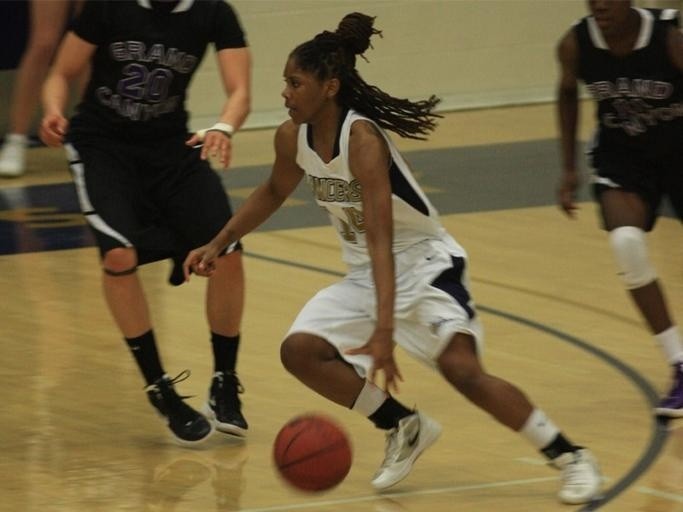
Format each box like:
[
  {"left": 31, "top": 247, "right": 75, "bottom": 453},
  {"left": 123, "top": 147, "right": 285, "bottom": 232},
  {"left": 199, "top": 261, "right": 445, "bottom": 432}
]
[
  {"left": 0, "top": 0, "right": 93, "bottom": 178},
  {"left": 37, "top": 0, "right": 252, "bottom": 445},
  {"left": 181, "top": 12, "right": 604, "bottom": 506},
  {"left": 555, "top": 0, "right": 683, "bottom": 418}
]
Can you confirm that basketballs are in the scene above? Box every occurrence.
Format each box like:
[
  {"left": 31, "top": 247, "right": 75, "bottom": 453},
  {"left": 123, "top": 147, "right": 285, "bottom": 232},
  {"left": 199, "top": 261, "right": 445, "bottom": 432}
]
[{"left": 274, "top": 418, "right": 352, "bottom": 491}]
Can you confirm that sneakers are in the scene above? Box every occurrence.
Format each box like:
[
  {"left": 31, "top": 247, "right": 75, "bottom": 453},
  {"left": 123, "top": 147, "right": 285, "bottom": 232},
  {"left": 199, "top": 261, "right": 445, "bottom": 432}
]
[
  {"left": 547, "top": 449, "right": 602, "bottom": 504},
  {"left": 0, "top": 134, "right": 26, "bottom": 176},
  {"left": 653, "top": 365, "right": 683, "bottom": 419},
  {"left": 142, "top": 370, "right": 212, "bottom": 444},
  {"left": 372, "top": 413, "right": 441, "bottom": 491},
  {"left": 207, "top": 372, "right": 252, "bottom": 437}
]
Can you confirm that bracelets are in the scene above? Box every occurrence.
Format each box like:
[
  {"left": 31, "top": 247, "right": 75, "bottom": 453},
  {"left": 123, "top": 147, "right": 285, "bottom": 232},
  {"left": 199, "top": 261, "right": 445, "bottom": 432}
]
[{"left": 195, "top": 123, "right": 234, "bottom": 143}]
